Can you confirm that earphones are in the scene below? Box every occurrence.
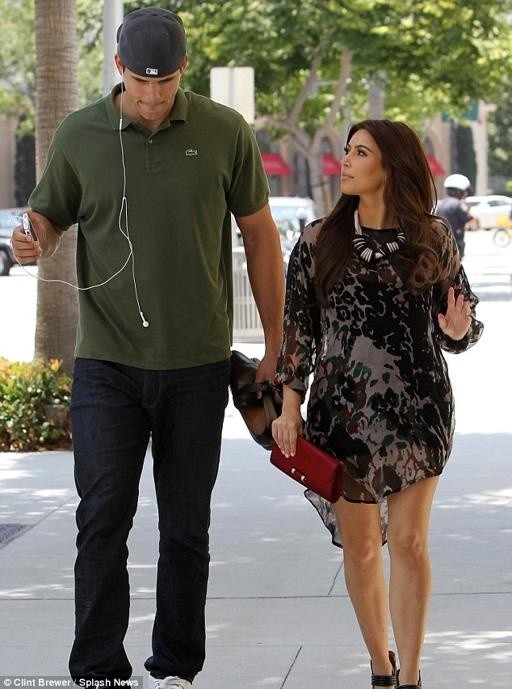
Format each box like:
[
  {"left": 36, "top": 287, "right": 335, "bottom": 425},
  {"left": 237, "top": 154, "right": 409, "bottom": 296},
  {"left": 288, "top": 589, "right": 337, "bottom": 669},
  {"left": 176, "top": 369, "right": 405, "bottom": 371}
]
[{"left": 140, "top": 312, "right": 150, "bottom": 328}]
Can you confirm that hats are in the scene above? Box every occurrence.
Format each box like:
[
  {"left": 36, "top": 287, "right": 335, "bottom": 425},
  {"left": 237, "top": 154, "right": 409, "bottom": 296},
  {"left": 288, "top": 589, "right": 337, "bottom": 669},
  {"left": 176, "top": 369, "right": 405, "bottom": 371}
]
[{"left": 117, "top": 5, "right": 188, "bottom": 79}]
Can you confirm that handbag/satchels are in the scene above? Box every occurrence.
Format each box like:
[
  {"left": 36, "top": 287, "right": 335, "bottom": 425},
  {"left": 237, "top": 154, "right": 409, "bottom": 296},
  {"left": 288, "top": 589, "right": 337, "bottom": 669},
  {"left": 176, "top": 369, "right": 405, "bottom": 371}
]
[{"left": 272, "top": 431, "right": 343, "bottom": 504}]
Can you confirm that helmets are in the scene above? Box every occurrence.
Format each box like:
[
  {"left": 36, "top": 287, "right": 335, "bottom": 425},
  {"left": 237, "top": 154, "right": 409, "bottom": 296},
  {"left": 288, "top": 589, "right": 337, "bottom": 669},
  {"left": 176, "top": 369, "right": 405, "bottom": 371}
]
[{"left": 444, "top": 173, "right": 470, "bottom": 199}]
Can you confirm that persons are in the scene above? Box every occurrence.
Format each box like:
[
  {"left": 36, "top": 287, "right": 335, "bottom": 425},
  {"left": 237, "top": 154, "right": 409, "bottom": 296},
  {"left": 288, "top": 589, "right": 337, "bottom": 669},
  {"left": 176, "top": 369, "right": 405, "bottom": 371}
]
[
  {"left": 433, "top": 173, "right": 478, "bottom": 264},
  {"left": 271, "top": 118, "right": 485, "bottom": 689},
  {"left": 8, "top": 5, "right": 287, "bottom": 689}
]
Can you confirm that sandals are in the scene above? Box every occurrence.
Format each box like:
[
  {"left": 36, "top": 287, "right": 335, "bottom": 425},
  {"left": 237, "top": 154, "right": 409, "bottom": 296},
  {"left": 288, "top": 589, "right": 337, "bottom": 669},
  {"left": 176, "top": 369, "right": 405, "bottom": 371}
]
[{"left": 369, "top": 651, "right": 421, "bottom": 689}]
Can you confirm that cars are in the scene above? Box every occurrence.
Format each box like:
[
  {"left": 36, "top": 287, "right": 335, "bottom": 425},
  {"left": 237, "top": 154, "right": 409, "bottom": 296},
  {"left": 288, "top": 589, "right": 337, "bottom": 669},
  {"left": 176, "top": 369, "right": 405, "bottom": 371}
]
[{"left": 465, "top": 194, "right": 512, "bottom": 232}]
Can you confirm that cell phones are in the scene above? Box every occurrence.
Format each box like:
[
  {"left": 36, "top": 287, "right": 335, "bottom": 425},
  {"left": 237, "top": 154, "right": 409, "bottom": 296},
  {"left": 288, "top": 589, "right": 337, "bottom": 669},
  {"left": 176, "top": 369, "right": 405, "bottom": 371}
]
[{"left": 23, "top": 214, "right": 40, "bottom": 247}]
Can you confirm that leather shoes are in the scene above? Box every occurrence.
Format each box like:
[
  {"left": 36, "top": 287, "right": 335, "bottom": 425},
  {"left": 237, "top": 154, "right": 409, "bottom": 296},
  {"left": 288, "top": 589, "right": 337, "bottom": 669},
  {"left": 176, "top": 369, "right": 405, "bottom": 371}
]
[{"left": 230, "top": 350, "right": 285, "bottom": 451}]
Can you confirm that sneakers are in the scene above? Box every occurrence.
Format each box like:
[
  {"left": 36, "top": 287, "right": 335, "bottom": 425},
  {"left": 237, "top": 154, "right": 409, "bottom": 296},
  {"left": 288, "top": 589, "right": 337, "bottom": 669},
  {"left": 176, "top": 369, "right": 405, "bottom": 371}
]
[{"left": 154, "top": 675, "right": 192, "bottom": 689}]
